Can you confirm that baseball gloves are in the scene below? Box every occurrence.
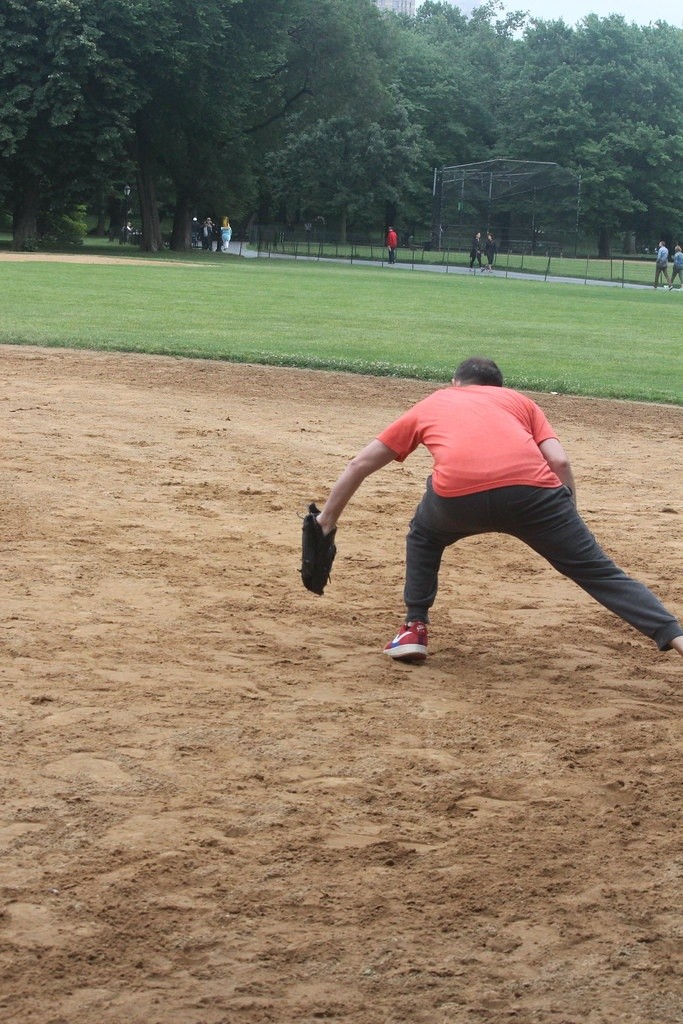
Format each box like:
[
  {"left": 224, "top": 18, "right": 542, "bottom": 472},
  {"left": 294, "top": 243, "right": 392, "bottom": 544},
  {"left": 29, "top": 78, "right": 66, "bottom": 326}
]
[{"left": 296, "top": 500, "right": 338, "bottom": 597}]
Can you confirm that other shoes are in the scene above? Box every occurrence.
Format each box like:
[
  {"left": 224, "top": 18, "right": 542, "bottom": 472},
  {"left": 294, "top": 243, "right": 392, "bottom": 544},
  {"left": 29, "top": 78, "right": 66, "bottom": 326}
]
[
  {"left": 469, "top": 268, "right": 475, "bottom": 272},
  {"left": 664, "top": 285, "right": 669, "bottom": 289},
  {"left": 678, "top": 285, "right": 683, "bottom": 290},
  {"left": 653, "top": 284, "right": 657, "bottom": 288},
  {"left": 486, "top": 268, "right": 492, "bottom": 273},
  {"left": 669, "top": 286, "right": 673, "bottom": 291},
  {"left": 480, "top": 267, "right": 485, "bottom": 272},
  {"left": 388, "top": 260, "right": 396, "bottom": 264}
]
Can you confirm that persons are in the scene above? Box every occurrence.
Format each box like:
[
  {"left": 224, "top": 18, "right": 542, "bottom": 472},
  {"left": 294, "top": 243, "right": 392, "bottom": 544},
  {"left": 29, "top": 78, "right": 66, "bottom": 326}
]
[
  {"left": 484, "top": 233, "right": 497, "bottom": 273},
  {"left": 653, "top": 241, "right": 673, "bottom": 290},
  {"left": 470, "top": 232, "right": 485, "bottom": 272},
  {"left": 199, "top": 217, "right": 232, "bottom": 251},
  {"left": 192, "top": 217, "right": 199, "bottom": 241},
  {"left": 316, "top": 357, "right": 683, "bottom": 660},
  {"left": 123, "top": 222, "right": 133, "bottom": 231},
  {"left": 671, "top": 246, "right": 683, "bottom": 285},
  {"left": 386, "top": 227, "right": 398, "bottom": 264}
]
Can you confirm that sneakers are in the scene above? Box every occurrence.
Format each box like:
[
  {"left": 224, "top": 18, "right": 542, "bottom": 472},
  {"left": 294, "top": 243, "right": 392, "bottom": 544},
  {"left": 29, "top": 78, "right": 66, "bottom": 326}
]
[{"left": 384, "top": 622, "right": 430, "bottom": 660}]
